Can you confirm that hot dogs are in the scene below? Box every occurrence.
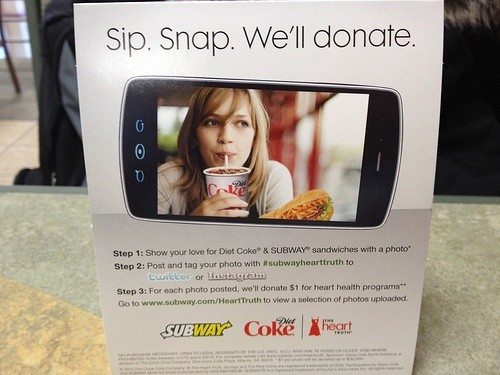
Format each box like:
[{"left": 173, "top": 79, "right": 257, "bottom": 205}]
[{"left": 259, "top": 190, "right": 333, "bottom": 220}]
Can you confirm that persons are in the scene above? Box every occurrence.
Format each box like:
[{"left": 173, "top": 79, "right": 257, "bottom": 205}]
[{"left": 157, "top": 86, "right": 295, "bottom": 220}]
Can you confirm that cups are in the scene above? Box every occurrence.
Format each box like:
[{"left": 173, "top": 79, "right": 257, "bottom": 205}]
[{"left": 203, "top": 166, "right": 251, "bottom": 211}]
